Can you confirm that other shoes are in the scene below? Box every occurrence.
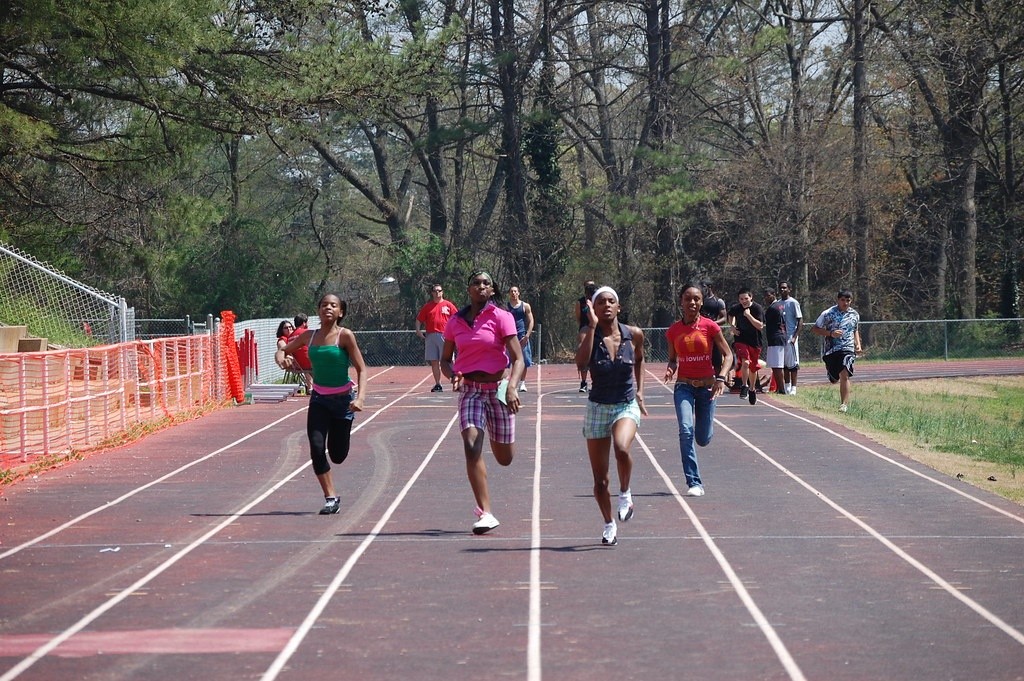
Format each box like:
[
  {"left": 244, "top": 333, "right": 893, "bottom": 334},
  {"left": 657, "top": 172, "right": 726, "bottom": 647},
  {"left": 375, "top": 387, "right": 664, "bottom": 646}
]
[
  {"left": 774, "top": 390, "right": 786, "bottom": 395},
  {"left": 756, "top": 385, "right": 762, "bottom": 394},
  {"left": 785, "top": 384, "right": 796, "bottom": 395},
  {"left": 729, "top": 384, "right": 742, "bottom": 394},
  {"left": 306, "top": 389, "right": 313, "bottom": 395}
]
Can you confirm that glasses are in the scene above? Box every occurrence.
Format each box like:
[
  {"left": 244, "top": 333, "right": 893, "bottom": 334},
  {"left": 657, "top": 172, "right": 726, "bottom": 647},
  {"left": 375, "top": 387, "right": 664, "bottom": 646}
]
[
  {"left": 283, "top": 326, "right": 292, "bottom": 329},
  {"left": 431, "top": 289, "right": 442, "bottom": 292},
  {"left": 469, "top": 279, "right": 491, "bottom": 286}
]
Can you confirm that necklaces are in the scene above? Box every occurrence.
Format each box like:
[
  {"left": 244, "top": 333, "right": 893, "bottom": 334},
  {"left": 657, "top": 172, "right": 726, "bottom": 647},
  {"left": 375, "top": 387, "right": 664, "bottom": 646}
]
[{"left": 682, "top": 313, "right": 700, "bottom": 325}]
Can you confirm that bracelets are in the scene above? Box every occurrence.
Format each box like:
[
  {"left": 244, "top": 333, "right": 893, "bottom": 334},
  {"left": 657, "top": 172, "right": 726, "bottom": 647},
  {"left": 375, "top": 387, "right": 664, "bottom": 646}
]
[
  {"left": 830, "top": 330, "right": 834, "bottom": 337},
  {"left": 587, "top": 325, "right": 595, "bottom": 329},
  {"left": 717, "top": 376, "right": 726, "bottom": 382}
]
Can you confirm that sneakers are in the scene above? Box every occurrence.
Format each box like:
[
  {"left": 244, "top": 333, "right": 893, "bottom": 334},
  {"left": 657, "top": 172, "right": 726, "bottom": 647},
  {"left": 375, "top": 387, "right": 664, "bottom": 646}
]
[
  {"left": 617, "top": 493, "right": 634, "bottom": 522},
  {"left": 739, "top": 385, "right": 750, "bottom": 399},
  {"left": 579, "top": 381, "right": 587, "bottom": 393},
  {"left": 686, "top": 486, "right": 705, "bottom": 497},
  {"left": 519, "top": 382, "right": 527, "bottom": 392},
  {"left": 602, "top": 524, "right": 618, "bottom": 546},
  {"left": 472, "top": 507, "right": 500, "bottom": 535},
  {"left": 320, "top": 496, "right": 341, "bottom": 515},
  {"left": 431, "top": 384, "right": 443, "bottom": 392},
  {"left": 837, "top": 404, "right": 848, "bottom": 413},
  {"left": 747, "top": 389, "right": 759, "bottom": 405}
]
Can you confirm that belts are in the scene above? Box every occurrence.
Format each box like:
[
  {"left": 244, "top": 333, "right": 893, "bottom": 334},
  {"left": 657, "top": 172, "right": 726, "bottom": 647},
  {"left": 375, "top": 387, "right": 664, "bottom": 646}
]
[
  {"left": 462, "top": 379, "right": 499, "bottom": 391},
  {"left": 676, "top": 375, "right": 716, "bottom": 388}
]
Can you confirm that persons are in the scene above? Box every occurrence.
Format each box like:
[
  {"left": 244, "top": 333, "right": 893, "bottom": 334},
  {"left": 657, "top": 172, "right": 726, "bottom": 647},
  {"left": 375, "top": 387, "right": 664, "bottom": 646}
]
[
  {"left": 576, "top": 285, "right": 648, "bottom": 546},
  {"left": 778, "top": 281, "right": 802, "bottom": 396},
  {"left": 506, "top": 286, "right": 534, "bottom": 392},
  {"left": 575, "top": 280, "right": 596, "bottom": 393},
  {"left": 811, "top": 289, "right": 862, "bottom": 412},
  {"left": 764, "top": 288, "right": 787, "bottom": 395},
  {"left": 440, "top": 270, "right": 525, "bottom": 535},
  {"left": 700, "top": 280, "right": 727, "bottom": 377},
  {"left": 416, "top": 284, "right": 458, "bottom": 392},
  {"left": 275, "top": 294, "right": 367, "bottom": 515},
  {"left": 286, "top": 314, "right": 313, "bottom": 395},
  {"left": 726, "top": 289, "right": 763, "bottom": 405},
  {"left": 277, "top": 321, "right": 294, "bottom": 354},
  {"left": 664, "top": 284, "right": 733, "bottom": 495}
]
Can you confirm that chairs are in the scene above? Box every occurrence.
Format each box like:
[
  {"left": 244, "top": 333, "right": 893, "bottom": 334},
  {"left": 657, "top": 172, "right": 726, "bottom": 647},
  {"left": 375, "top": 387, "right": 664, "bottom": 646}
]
[
  {"left": 285, "top": 356, "right": 313, "bottom": 394},
  {"left": 282, "top": 357, "right": 297, "bottom": 384}
]
